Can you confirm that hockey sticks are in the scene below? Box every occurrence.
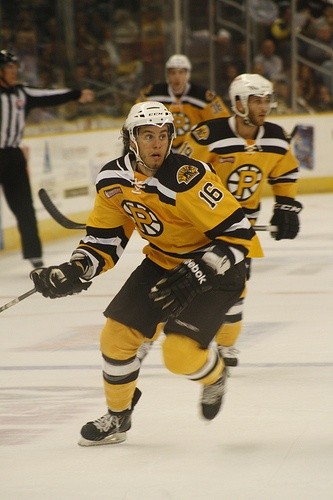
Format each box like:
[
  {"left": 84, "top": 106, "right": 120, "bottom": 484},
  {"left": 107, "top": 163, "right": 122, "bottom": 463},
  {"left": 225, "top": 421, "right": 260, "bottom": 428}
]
[
  {"left": 37, "top": 188, "right": 278, "bottom": 233},
  {"left": 0, "top": 288, "right": 39, "bottom": 313}
]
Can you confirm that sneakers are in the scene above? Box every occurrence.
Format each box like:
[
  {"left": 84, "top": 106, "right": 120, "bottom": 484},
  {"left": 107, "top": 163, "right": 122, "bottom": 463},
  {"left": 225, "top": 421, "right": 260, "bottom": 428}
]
[
  {"left": 217, "top": 344, "right": 238, "bottom": 366},
  {"left": 200, "top": 367, "right": 226, "bottom": 420},
  {"left": 79, "top": 387, "right": 142, "bottom": 446}
]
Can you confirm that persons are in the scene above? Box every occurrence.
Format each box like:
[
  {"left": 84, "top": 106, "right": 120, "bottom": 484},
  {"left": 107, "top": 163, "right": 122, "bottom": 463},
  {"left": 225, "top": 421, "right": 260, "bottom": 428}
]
[
  {"left": 0, "top": 50, "right": 94, "bottom": 279},
  {"left": 136, "top": 74, "right": 302, "bottom": 376},
  {"left": 0, "top": 0, "right": 333, "bottom": 123},
  {"left": 135, "top": 55, "right": 230, "bottom": 151},
  {"left": 26, "top": 102, "right": 264, "bottom": 447}
]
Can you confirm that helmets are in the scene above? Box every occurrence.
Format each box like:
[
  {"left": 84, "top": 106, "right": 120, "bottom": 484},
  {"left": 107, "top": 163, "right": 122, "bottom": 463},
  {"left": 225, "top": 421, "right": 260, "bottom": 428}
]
[
  {"left": 124, "top": 101, "right": 175, "bottom": 130},
  {"left": 229, "top": 74, "right": 274, "bottom": 117},
  {"left": 165, "top": 54, "right": 192, "bottom": 70}
]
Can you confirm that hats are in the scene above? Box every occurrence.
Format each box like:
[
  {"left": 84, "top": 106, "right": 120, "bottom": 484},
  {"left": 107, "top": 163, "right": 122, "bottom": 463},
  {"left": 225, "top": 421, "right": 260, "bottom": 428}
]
[{"left": 0, "top": 49, "right": 18, "bottom": 64}]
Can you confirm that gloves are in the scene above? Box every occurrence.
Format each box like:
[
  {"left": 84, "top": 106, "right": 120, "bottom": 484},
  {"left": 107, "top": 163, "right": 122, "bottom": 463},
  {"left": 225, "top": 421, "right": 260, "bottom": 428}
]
[
  {"left": 270, "top": 196, "right": 303, "bottom": 240},
  {"left": 149, "top": 259, "right": 213, "bottom": 317},
  {"left": 29, "top": 259, "right": 92, "bottom": 299}
]
